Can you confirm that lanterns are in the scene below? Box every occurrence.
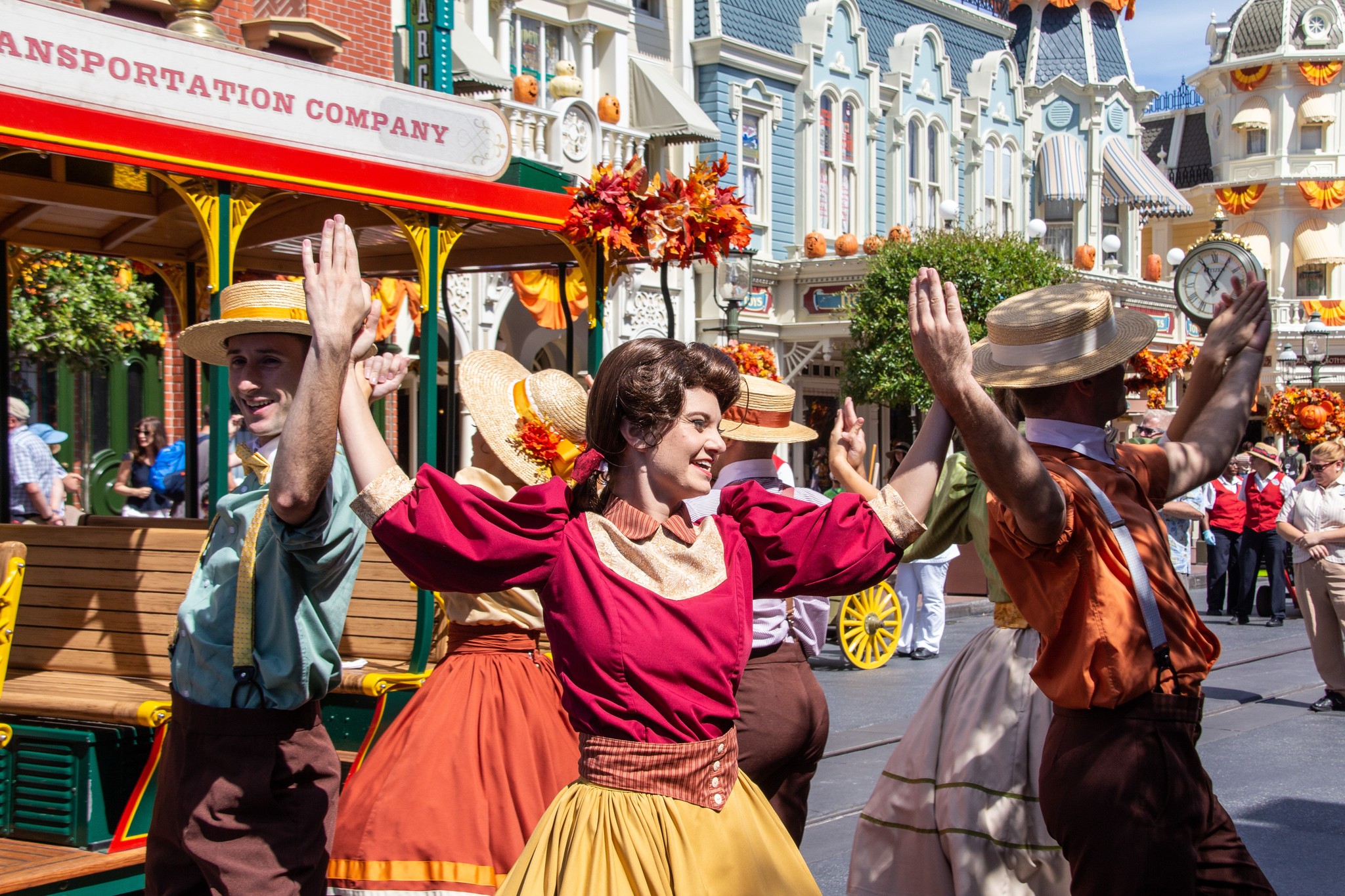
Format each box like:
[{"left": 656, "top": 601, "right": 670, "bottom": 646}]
[
  {"left": 514, "top": 59, "right": 621, "bottom": 124},
  {"left": 1073, "top": 243, "right": 1096, "bottom": 271},
  {"left": 805, "top": 224, "right": 911, "bottom": 260},
  {"left": 1145, "top": 253, "right": 1162, "bottom": 283}
]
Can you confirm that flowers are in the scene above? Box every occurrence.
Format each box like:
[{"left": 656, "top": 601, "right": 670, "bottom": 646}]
[
  {"left": 6, "top": 243, "right": 166, "bottom": 368},
  {"left": 1122, "top": 344, "right": 1200, "bottom": 410},
  {"left": 1266, "top": 387, "right": 1345, "bottom": 445},
  {"left": 562, "top": 151, "right": 755, "bottom": 271},
  {"left": 508, "top": 409, "right": 563, "bottom": 480},
  {"left": 714, "top": 339, "right": 783, "bottom": 384}
]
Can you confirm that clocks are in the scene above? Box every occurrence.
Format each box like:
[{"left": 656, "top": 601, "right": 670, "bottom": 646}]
[{"left": 1174, "top": 241, "right": 1259, "bottom": 327}]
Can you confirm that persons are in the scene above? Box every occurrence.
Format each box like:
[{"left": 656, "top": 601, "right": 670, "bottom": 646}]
[
  {"left": 1226, "top": 441, "right": 1296, "bottom": 627},
  {"left": 823, "top": 442, "right": 962, "bottom": 659},
  {"left": 1137, "top": 408, "right": 1205, "bottom": 594},
  {"left": 113, "top": 416, "right": 172, "bottom": 519},
  {"left": 826, "top": 278, "right": 1270, "bottom": 896},
  {"left": 908, "top": 266, "right": 1276, "bottom": 896},
  {"left": 28, "top": 423, "right": 85, "bottom": 516},
  {"left": 1263, "top": 435, "right": 1279, "bottom": 464},
  {"left": 7, "top": 396, "right": 67, "bottom": 526},
  {"left": 171, "top": 395, "right": 260, "bottom": 519},
  {"left": 1274, "top": 437, "right": 1345, "bottom": 711},
  {"left": 325, "top": 299, "right": 595, "bottom": 895},
  {"left": 1278, "top": 439, "right": 1308, "bottom": 486},
  {"left": 1235, "top": 442, "right": 1253, "bottom": 479},
  {"left": 147, "top": 212, "right": 410, "bottom": 896},
  {"left": 681, "top": 373, "right": 868, "bottom": 849},
  {"left": 1201, "top": 455, "right": 1247, "bottom": 615}
]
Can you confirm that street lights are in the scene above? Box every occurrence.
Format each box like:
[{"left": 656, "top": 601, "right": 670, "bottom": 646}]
[
  {"left": 1276, "top": 343, "right": 1300, "bottom": 450},
  {"left": 703, "top": 244, "right": 763, "bottom": 348},
  {"left": 1295, "top": 309, "right": 1337, "bottom": 481}
]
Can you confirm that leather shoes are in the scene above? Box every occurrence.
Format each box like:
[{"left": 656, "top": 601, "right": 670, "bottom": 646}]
[
  {"left": 1266, "top": 616, "right": 1283, "bottom": 627},
  {"left": 1310, "top": 693, "right": 1345, "bottom": 711},
  {"left": 880, "top": 649, "right": 913, "bottom": 658},
  {"left": 1227, "top": 615, "right": 1250, "bottom": 625},
  {"left": 910, "top": 647, "right": 939, "bottom": 659}
]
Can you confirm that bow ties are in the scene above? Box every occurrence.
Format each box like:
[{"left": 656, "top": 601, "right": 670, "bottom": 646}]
[
  {"left": 235, "top": 442, "right": 270, "bottom": 486},
  {"left": 1105, "top": 427, "right": 1121, "bottom": 462}
]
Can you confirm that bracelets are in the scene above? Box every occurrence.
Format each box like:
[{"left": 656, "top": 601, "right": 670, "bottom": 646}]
[
  {"left": 52, "top": 509, "right": 60, "bottom": 514},
  {"left": 43, "top": 514, "right": 53, "bottom": 521}
]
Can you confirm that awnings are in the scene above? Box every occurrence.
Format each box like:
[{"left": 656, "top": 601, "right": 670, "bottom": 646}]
[
  {"left": 628, "top": 59, "right": 721, "bottom": 146},
  {"left": 452, "top": 16, "right": 513, "bottom": 97},
  {"left": 1102, "top": 138, "right": 1194, "bottom": 220},
  {"left": 1037, "top": 134, "right": 1088, "bottom": 205}
]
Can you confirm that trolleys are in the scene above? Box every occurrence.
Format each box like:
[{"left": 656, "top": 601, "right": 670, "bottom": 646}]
[{"left": 827, "top": 581, "right": 903, "bottom": 669}]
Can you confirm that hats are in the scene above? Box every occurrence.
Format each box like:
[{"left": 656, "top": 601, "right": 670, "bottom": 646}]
[
  {"left": 885, "top": 442, "right": 911, "bottom": 460},
  {"left": 830, "top": 472, "right": 838, "bottom": 480},
  {"left": 28, "top": 422, "right": 69, "bottom": 445},
  {"left": 8, "top": 396, "right": 30, "bottom": 420},
  {"left": 971, "top": 283, "right": 1158, "bottom": 388},
  {"left": 176, "top": 280, "right": 378, "bottom": 366},
  {"left": 1246, "top": 443, "right": 1280, "bottom": 467},
  {"left": 719, "top": 374, "right": 819, "bottom": 444},
  {"left": 458, "top": 349, "right": 588, "bottom": 486}
]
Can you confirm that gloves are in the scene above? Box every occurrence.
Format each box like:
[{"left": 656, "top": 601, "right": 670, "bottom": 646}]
[{"left": 1202, "top": 529, "right": 1216, "bottom": 546}]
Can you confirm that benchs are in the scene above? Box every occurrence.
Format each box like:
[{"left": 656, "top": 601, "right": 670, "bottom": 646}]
[{"left": 0, "top": 514, "right": 441, "bottom": 858}]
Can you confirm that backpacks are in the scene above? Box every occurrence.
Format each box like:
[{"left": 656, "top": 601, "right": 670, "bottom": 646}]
[
  {"left": 1282, "top": 451, "right": 1301, "bottom": 481},
  {"left": 147, "top": 434, "right": 210, "bottom": 502}
]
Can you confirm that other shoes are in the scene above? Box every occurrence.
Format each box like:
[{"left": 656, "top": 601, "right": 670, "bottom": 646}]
[{"left": 1206, "top": 608, "right": 1223, "bottom": 616}]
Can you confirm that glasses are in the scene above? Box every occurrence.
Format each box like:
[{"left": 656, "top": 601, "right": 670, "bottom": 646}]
[
  {"left": 1137, "top": 426, "right": 1166, "bottom": 435},
  {"left": 135, "top": 428, "right": 153, "bottom": 436},
  {"left": 1307, "top": 460, "right": 1338, "bottom": 472}
]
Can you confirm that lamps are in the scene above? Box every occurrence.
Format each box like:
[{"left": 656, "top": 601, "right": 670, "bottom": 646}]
[
  {"left": 1103, "top": 233, "right": 1121, "bottom": 260},
  {"left": 1027, "top": 218, "right": 1047, "bottom": 244},
  {"left": 1167, "top": 247, "right": 1186, "bottom": 270},
  {"left": 939, "top": 200, "right": 958, "bottom": 228}
]
